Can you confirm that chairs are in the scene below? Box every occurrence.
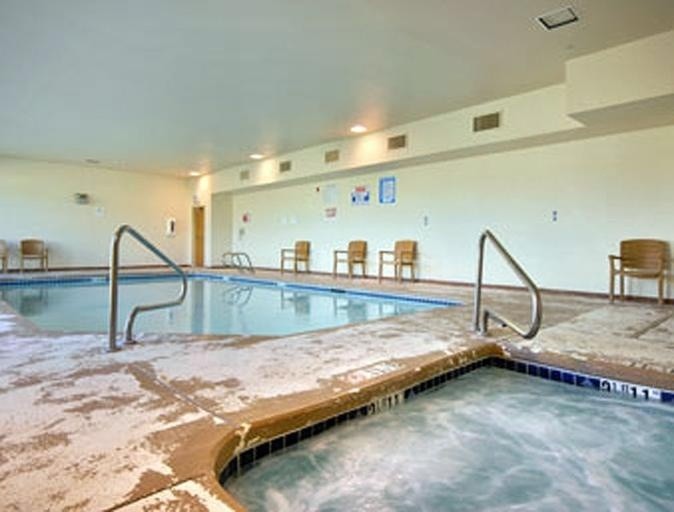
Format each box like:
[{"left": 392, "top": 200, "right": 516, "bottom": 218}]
[
  {"left": 334, "top": 240, "right": 366, "bottom": 279},
  {"left": 608, "top": 240, "right": 666, "bottom": 305},
  {"left": 0, "top": 239, "right": 48, "bottom": 274},
  {"left": 281, "top": 241, "right": 310, "bottom": 273},
  {"left": 379, "top": 241, "right": 416, "bottom": 283}
]
